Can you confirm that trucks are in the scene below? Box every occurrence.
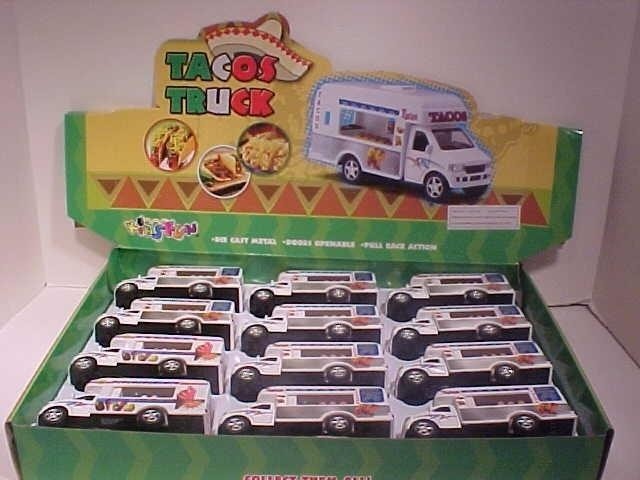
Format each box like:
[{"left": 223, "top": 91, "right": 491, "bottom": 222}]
[{"left": 308, "top": 81, "right": 494, "bottom": 201}]
